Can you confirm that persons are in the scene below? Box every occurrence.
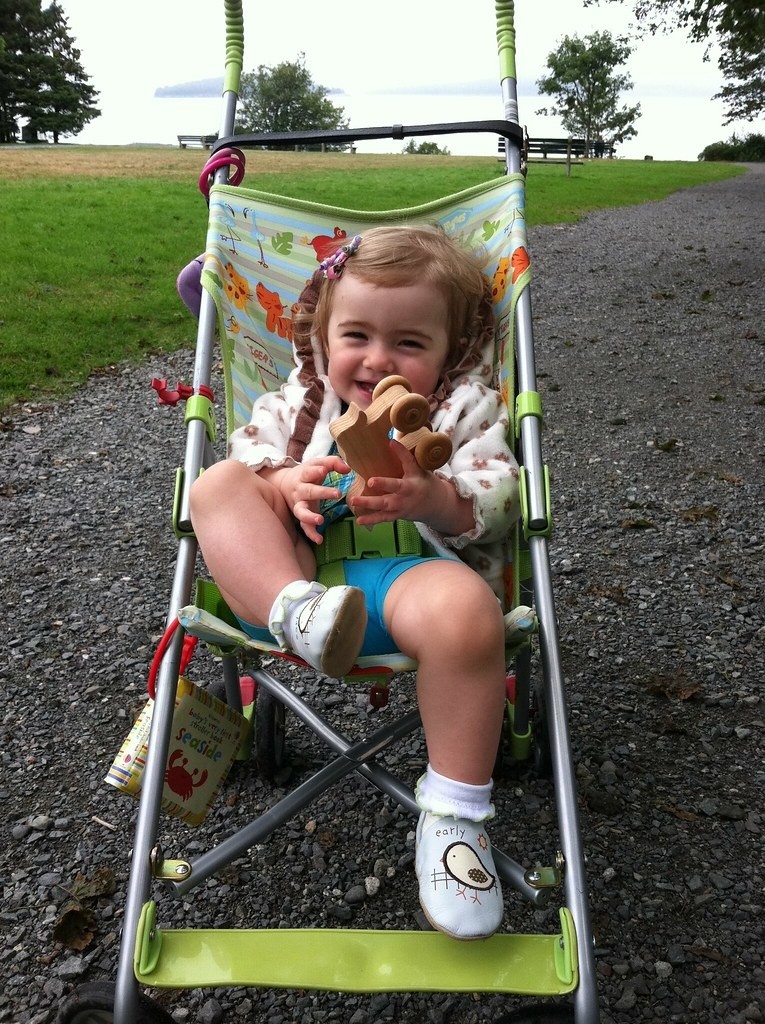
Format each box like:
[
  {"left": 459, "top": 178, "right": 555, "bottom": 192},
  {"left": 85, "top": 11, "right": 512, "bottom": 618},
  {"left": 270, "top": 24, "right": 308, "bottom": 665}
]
[{"left": 187, "top": 224, "right": 523, "bottom": 941}]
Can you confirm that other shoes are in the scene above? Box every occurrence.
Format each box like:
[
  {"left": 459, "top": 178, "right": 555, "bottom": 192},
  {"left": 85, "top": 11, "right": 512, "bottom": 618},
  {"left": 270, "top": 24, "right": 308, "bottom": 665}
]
[
  {"left": 415, "top": 809, "right": 504, "bottom": 941},
  {"left": 292, "top": 584, "right": 367, "bottom": 678}
]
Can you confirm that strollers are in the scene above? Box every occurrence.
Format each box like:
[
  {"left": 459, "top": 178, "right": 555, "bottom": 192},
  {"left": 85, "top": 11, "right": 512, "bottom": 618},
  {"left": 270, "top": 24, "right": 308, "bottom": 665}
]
[{"left": 55, "top": 0, "right": 598, "bottom": 1024}]
